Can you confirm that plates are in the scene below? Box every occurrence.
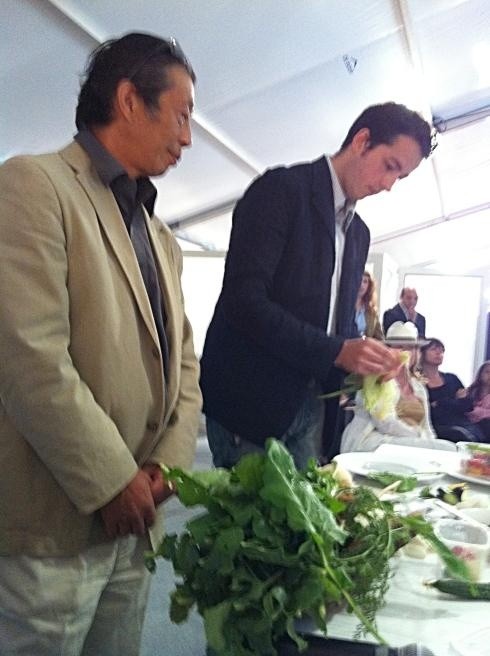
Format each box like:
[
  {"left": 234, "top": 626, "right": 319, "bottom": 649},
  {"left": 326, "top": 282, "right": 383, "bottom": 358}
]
[
  {"left": 444, "top": 450, "right": 490, "bottom": 486},
  {"left": 332, "top": 451, "right": 446, "bottom": 486}
]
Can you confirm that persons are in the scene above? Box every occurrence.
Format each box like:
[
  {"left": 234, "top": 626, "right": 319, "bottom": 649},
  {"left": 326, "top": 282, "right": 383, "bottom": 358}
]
[
  {"left": 1, "top": 31, "right": 201, "bottom": 655},
  {"left": 198, "top": 101, "right": 432, "bottom": 484},
  {"left": 333, "top": 272, "right": 489, "bottom": 453}
]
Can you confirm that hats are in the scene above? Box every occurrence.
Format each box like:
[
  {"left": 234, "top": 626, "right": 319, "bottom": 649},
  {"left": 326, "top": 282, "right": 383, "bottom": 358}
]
[{"left": 384, "top": 320, "right": 431, "bottom": 346}]
